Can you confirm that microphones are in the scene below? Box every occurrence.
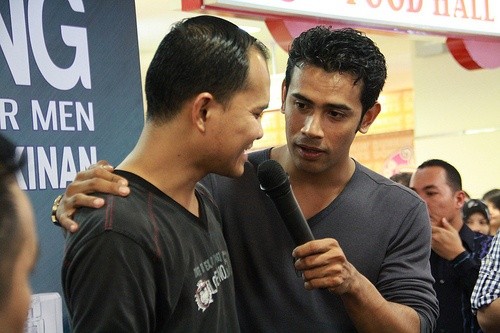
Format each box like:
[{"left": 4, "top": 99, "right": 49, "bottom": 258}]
[{"left": 258, "top": 159, "right": 315, "bottom": 247}]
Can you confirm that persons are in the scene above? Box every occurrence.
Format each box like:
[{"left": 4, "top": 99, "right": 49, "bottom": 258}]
[
  {"left": 409, "top": 159, "right": 494, "bottom": 333},
  {"left": 0, "top": 133, "right": 38, "bottom": 333},
  {"left": 52, "top": 23, "right": 439, "bottom": 333},
  {"left": 481, "top": 189, "right": 500, "bottom": 237},
  {"left": 389, "top": 171, "right": 412, "bottom": 187},
  {"left": 471, "top": 224, "right": 500, "bottom": 333},
  {"left": 61, "top": 15, "right": 269, "bottom": 333},
  {"left": 462, "top": 198, "right": 491, "bottom": 235}
]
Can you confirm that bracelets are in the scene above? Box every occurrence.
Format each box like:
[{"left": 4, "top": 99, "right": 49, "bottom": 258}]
[{"left": 52, "top": 192, "right": 62, "bottom": 223}]
